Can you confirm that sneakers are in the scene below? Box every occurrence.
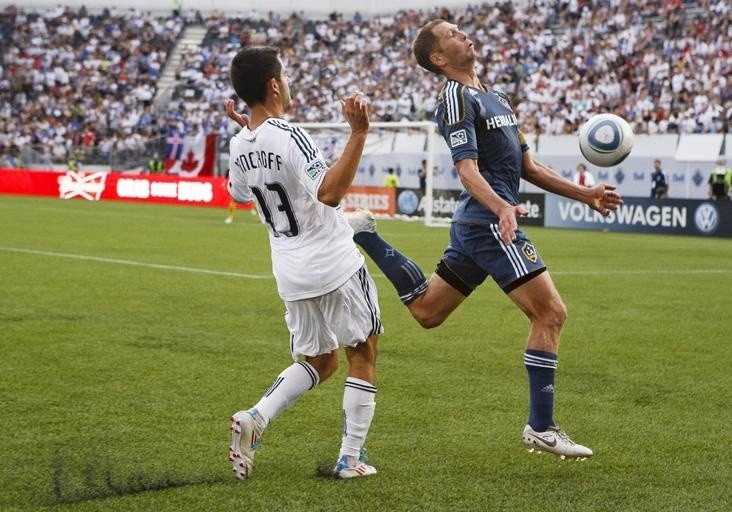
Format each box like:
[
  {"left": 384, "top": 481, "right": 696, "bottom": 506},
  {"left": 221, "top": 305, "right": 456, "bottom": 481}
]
[
  {"left": 228, "top": 409, "right": 261, "bottom": 480},
  {"left": 332, "top": 461, "right": 378, "bottom": 479},
  {"left": 343, "top": 209, "right": 377, "bottom": 235},
  {"left": 522, "top": 422, "right": 593, "bottom": 462}
]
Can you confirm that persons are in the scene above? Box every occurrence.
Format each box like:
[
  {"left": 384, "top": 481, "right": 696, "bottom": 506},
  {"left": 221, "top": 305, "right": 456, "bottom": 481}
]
[
  {"left": 347, "top": 18, "right": 623, "bottom": 458},
  {"left": 225, "top": 47, "right": 383, "bottom": 482}
]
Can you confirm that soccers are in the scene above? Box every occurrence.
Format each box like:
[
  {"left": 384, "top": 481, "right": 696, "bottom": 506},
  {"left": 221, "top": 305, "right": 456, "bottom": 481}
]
[{"left": 579, "top": 114, "right": 634, "bottom": 167}]
